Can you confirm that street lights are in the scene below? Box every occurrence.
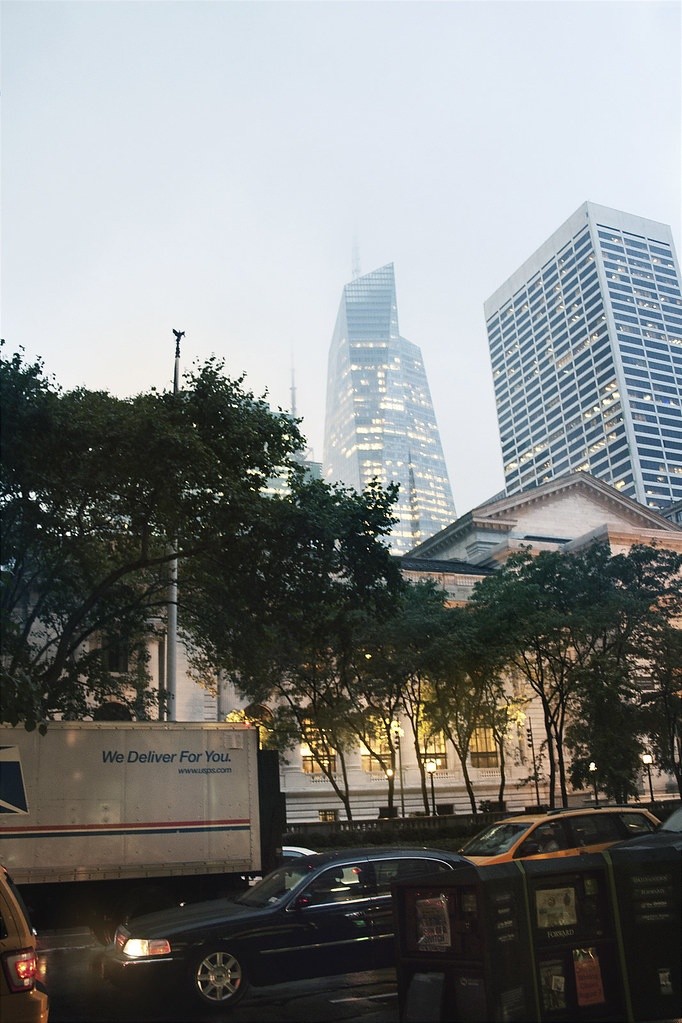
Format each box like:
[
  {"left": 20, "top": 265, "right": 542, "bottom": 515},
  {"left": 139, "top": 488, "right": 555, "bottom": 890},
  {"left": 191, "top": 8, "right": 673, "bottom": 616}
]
[
  {"left": 589, "top": 762, "right": 598, "bottom": 805},
  {"left": 427, "top": 761, "right": 438, "bottom": 816},
  {"left": 643, "top": 754, "right": 655, "bottom": 801}
]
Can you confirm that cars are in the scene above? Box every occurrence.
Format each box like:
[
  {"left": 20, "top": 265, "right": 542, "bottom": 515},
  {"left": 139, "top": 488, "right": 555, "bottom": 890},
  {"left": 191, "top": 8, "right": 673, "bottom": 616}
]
[
  {"left": 1, "top": 863, "right": 49, "bottom": 1023},
  {"left": 607, "top": 801, "right": 682, "bottom": 871},
  {"left": 242, "top": 847, "right": 359, "bottom": 892},
  {"left": 99, "top": 847, "right": 502, "bottom": 1009}
]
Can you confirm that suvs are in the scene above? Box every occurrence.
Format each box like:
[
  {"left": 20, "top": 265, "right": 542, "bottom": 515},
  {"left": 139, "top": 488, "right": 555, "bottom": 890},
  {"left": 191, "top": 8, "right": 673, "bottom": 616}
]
[{"left": 457, "top": 807, "right": 662, "bottom": 927}]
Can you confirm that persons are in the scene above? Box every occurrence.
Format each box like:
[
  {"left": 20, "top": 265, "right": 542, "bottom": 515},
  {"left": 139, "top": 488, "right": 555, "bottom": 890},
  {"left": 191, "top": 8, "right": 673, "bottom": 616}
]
[{"left": 536, "top": 829, "right": 558, "bottom": 853}]
